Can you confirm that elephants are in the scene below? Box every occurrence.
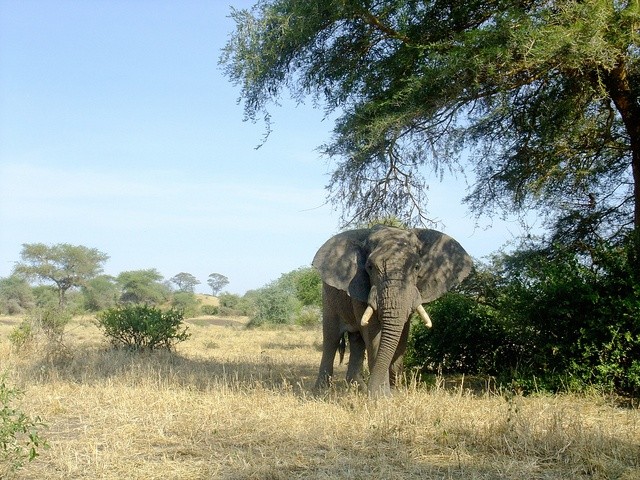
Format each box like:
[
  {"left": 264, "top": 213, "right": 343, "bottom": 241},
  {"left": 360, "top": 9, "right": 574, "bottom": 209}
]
[{"left": 307, "top": 223, "right": 473, "bottom": 401}]
